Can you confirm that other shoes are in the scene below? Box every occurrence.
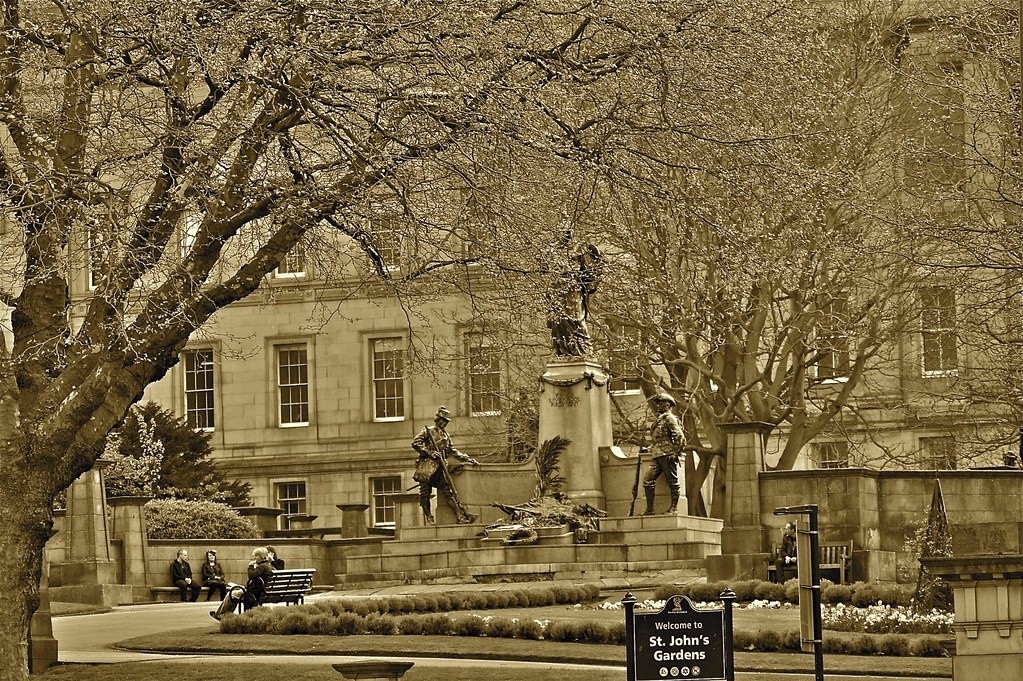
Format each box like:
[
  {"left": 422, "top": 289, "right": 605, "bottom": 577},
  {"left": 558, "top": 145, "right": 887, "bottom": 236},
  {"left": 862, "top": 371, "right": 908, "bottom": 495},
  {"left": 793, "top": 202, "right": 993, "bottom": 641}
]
[{"left": 210, "top": 611, "right": 221, "bottom": 621}]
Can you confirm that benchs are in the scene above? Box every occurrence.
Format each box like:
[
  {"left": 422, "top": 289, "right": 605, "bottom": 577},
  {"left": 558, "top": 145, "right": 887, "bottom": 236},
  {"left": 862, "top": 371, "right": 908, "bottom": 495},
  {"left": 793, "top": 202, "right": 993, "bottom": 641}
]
[
  {"left": 767, "top": 539, "right": 854, "bottom": 585},
  {"left": 150, "top": 586, "right": 230, "bottom": 600},
  {"left": 229, "top": 568, "right": 316, "bottom": 615}
]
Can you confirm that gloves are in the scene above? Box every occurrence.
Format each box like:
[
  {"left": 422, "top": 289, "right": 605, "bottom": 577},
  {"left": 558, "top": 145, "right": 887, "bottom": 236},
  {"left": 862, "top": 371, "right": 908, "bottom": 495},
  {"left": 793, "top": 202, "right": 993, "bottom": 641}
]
[{"left": 785, "top": 556, "right": 796, "bottom": 564}]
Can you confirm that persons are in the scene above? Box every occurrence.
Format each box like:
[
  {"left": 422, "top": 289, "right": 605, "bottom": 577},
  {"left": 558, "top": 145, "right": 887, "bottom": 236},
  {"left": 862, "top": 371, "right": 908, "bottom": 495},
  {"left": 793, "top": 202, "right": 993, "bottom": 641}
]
[
  {"left": 209, "top": 545, "right": 285, "bottom": 622},
  {"left": 635, "top": 393, "right": 687, "bottom": 516},
  {"left": 775, "top": 522, "right": 797, "bottom": 583},
  {"left": 170, "top": 549, "right": 201, "bottom": 603},
  {"left": 411, "top": 405, "right": 479, "bottom": 524},
  {"left": 540, "top": 220, "right": 596, "bottom": 356},
  {"left": 202, "top": 550, "right": 226, "bottom": 602}
]
